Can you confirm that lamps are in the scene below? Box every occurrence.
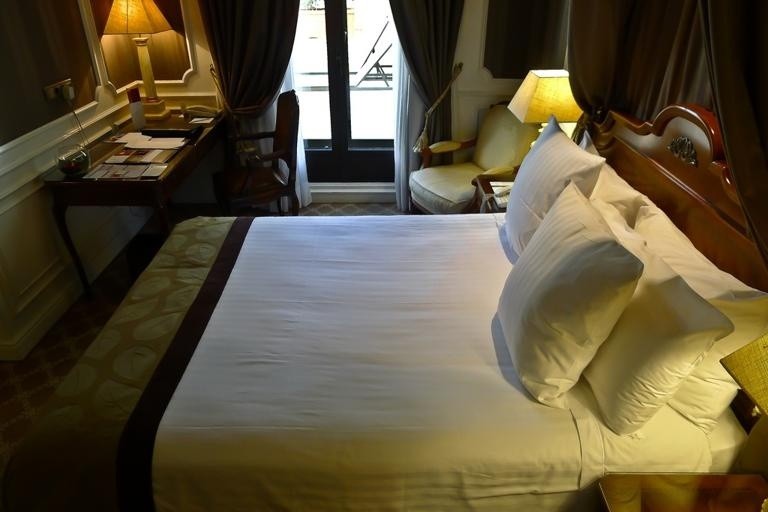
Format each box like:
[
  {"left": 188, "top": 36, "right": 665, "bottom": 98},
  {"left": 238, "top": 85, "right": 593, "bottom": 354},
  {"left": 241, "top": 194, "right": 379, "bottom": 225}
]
[
  {"left": 507, "top": 68, "right": 583, "bottom": 147},
  {"left": 103, "top": 0, "right": 174, "bottom": 119}
]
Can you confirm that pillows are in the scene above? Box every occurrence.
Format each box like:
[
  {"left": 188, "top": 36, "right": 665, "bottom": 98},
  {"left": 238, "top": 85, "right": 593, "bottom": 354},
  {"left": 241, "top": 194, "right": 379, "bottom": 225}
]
[{"left": 498, "top": 114, "right": 767, "bottom": 436}]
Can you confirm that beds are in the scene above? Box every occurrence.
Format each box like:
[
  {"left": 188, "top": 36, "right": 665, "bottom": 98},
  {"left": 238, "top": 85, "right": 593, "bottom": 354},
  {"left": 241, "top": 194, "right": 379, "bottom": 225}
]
[{"left": 1, "top": 104, "right": 765, "bottom": 512}]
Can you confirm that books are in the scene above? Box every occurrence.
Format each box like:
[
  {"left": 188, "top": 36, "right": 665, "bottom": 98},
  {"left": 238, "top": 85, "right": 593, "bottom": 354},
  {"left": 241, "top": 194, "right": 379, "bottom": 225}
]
[{"left": 81, "top": 116, "right": 215, "bottom": 182}]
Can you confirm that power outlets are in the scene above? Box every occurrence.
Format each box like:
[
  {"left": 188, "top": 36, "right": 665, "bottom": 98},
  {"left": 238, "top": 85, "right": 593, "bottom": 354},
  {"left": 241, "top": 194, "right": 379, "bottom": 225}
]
[{"left": 43, "top": 77, "right": 73, "bottom": 100}]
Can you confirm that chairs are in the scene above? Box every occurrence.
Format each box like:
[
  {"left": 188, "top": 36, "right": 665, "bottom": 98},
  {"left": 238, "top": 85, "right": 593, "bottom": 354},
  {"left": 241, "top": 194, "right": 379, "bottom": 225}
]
[
  {"left": 295, "top": 15, "right": 392, "bottom": 91},
  {"left": 407, "top": 100, "right": 542, "bottom": 214},
  {"left": 212, "top": 89, "right": 301, "bottom": 215}
]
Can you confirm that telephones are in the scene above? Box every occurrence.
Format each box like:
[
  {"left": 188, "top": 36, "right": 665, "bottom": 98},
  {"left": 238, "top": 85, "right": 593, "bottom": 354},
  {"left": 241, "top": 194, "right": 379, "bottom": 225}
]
[
  {"left": 182, "top": 105, "right": 223, "bottom": 122},
  {"left": 494, "top": 185, "right": 512, "bottom": 208}
]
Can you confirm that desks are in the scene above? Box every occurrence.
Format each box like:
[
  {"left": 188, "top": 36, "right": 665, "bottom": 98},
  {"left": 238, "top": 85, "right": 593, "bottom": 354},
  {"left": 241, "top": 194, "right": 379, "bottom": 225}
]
[
  {"left": 41, "top": 110, "right": 231, "bottom": 299},
  {"left": 598, "top": 474, "right": 767, "bottom": 512},
  {"left": 476, "top": 174, "right": 516, "bottom": 212}
]
